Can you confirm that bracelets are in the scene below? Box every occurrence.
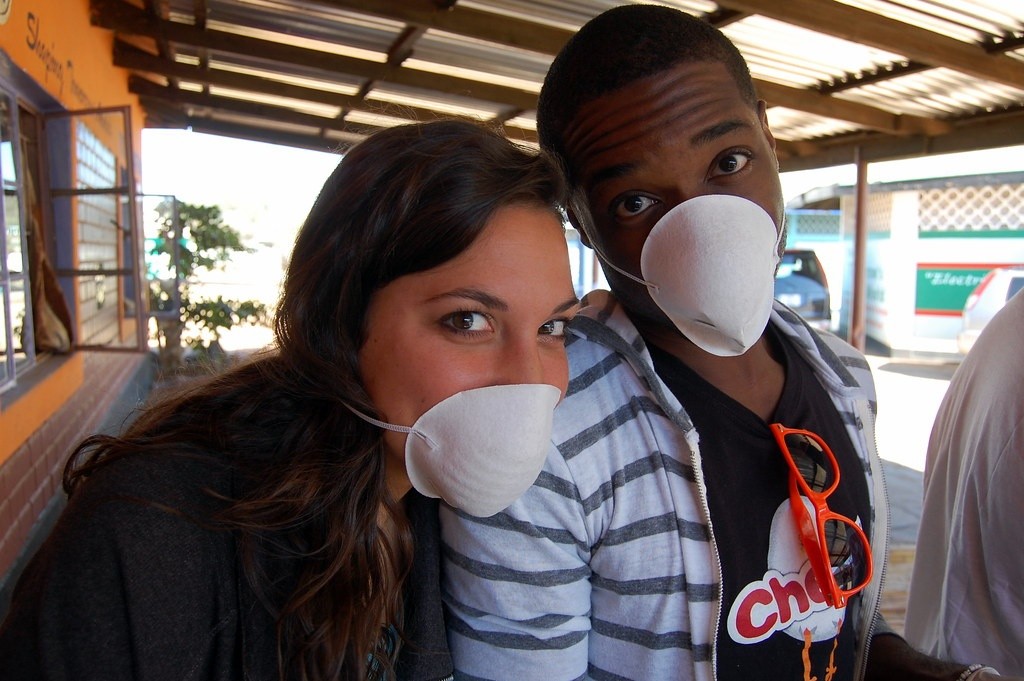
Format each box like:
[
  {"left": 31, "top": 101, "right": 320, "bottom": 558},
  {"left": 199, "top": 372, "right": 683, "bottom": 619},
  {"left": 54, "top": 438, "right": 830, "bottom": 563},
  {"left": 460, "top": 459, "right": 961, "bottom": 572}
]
[{"left": 956, "top": 663, "right": 1000, "bottom": 681}]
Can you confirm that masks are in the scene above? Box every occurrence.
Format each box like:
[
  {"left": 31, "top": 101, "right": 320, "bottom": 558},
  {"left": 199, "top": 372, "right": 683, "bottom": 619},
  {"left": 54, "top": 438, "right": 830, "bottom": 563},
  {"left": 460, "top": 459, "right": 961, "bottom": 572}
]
[
  {"left": 340, "top": 384, "right": 562, "bottom": 518},
  {"left": 576, "top": 192, "right": 786, "bottom": 357}
]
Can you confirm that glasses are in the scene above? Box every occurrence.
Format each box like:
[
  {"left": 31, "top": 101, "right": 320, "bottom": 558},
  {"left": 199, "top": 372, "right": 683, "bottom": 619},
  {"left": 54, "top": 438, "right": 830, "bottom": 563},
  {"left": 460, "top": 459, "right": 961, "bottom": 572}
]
[{"left": 770, "top": 423, "right": 873, "bottom": 609}]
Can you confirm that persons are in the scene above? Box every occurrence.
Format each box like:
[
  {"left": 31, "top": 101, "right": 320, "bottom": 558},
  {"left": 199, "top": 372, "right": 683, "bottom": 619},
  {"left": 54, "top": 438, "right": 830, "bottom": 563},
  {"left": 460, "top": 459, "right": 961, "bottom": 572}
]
[
  {"left": 904, "top": 289, "right": 1024, "bottom": 678},
  {"left": 439, "top": 5, "right": 1024, "bottom": 681},
  {"left": 0, "top": 120, "right": 582, "bottom": 681}
]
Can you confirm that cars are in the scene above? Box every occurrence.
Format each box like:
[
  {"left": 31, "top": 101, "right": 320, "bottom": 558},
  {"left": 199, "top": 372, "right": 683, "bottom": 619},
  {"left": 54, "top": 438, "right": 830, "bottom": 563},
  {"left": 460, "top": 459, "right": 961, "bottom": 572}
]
[{"left": 774, "top": 250, "right": 831, "bottom": 331}]
